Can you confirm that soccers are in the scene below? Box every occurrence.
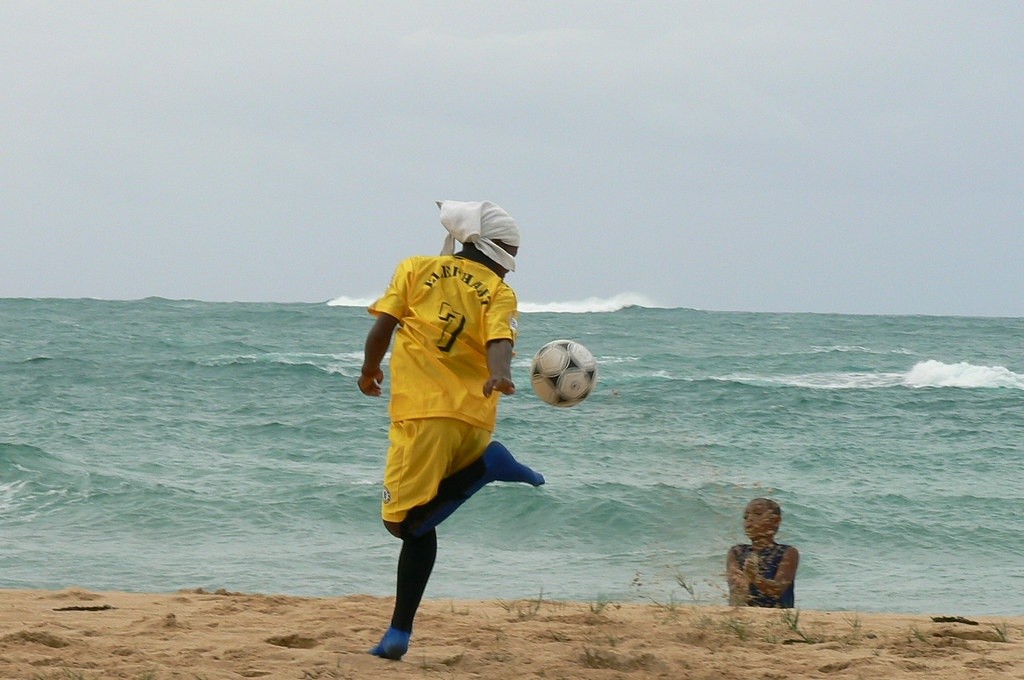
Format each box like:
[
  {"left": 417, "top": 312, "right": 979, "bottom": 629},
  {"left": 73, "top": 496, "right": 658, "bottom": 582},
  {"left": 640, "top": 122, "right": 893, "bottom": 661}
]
[{"left": 529, "top": 339, "right": 597, "bottom": 408}]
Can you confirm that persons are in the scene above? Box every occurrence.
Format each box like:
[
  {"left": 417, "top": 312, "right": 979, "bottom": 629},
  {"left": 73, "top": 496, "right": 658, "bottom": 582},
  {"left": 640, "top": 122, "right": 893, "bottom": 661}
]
[
  {"left": 356, "top": 200, "right": 546, "bottom": 661},
  {"left": 724, "top": 498, "right": 800, "bottom": 612}
]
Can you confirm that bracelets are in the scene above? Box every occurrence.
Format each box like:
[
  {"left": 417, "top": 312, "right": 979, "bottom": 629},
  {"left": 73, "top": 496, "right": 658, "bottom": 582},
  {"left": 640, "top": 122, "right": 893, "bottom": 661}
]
[{"left": 361, "top": 365, "right": 379, "bottom": 377}]
